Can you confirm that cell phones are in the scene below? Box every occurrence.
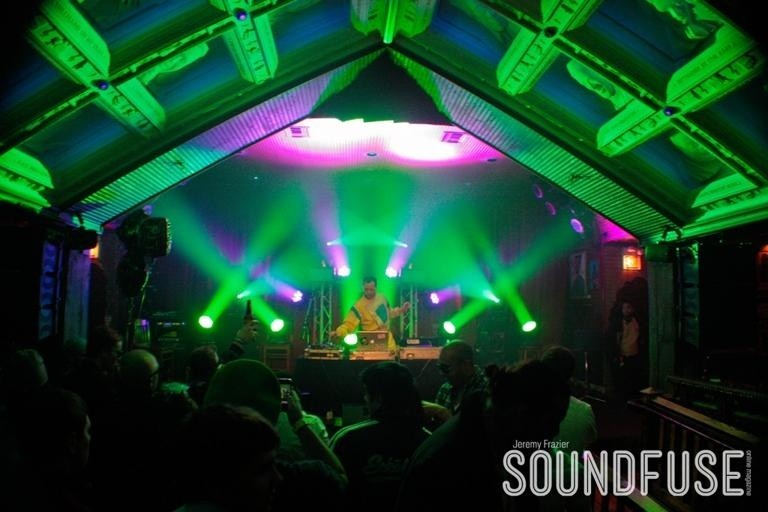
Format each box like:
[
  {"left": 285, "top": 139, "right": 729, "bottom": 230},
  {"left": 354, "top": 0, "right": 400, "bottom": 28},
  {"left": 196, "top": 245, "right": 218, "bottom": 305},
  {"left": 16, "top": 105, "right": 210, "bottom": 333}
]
[{"left": 277, "top": 377, "right": 293, "bottom": 411}]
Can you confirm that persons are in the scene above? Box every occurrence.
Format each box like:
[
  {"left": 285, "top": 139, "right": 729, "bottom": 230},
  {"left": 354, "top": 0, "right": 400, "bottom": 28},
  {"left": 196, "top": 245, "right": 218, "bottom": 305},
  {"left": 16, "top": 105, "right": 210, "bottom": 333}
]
[
  {"left": 184, "top": 319, "right": 262, "bottom": 408},
  {"left": 0, "top": 326, "right": 600, "bottom": 511},
  {"left": 617, "top": 300, "right": 643, "bottom": 358},
  {"left": 328, "top": 275, "right": 413, "bottom": 349}
]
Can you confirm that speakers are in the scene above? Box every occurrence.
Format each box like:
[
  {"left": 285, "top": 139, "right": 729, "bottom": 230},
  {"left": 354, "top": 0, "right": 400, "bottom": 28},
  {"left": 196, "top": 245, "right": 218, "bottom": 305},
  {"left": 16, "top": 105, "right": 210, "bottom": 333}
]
[{"left": 140, "top": 217, "right": 170, "bottom": 258}]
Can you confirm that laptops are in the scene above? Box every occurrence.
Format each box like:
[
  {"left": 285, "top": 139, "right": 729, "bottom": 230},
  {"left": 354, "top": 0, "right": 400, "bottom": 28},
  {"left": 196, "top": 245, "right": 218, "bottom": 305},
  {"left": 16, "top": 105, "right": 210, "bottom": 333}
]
[{"left": 356, "top": 331, "right": 390, "bottom": 352}]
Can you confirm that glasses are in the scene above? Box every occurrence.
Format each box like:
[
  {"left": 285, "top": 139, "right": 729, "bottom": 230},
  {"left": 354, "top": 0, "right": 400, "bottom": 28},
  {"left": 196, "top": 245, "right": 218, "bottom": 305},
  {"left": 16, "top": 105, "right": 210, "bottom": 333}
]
[{"left": 436, "top": 362, "right": 461, "bottom": 374}]
